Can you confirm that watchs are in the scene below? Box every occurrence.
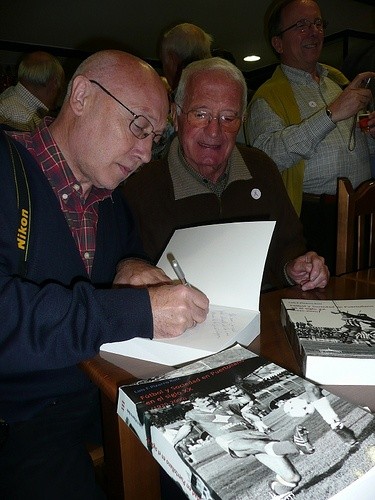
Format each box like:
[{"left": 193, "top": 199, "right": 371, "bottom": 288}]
[{"left": 326, "top": 108, "right": 336, "bottom": 124}]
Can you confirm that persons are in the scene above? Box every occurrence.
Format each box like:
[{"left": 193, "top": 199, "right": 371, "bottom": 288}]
[
  {"left": 160, "top": 23, "right": 237, "bottom": 113},
  {"left": 170, "top": 367, "right": 357, "bottom": 500},
  {"left": 110, "top": 59, "right": 331, "bottom": 292},
  {"left": 0, "top": 51, "right": 64, "bottom": 133},
  {"left": 242, "top": 0, "right": 375, "bottom": 276},
  {"left": 0, "top": 51, "right": 207, "bottom": 500}
]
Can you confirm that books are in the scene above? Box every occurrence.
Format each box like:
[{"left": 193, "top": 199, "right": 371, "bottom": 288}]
[
  {"left": 100, "top": 221, "right": 277, "bottom": 369},
  {"left": 279, "top": 298, "right": 375, "bottom": 388},
  {"left": 115, "top": 342, "right": 375, "bottom": 500}
]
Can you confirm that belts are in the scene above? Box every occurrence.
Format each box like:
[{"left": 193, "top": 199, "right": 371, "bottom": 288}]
[{"left": 301, "top": 193, "right": 337, "bottom": 204}]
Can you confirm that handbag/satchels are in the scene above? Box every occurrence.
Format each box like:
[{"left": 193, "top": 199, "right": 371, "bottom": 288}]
[{"left": 0, "top": 363, "right": 103, "bottom": 500}]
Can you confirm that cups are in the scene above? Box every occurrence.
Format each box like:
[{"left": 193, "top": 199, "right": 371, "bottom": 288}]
[{"left": 358, "top": 113, "right": 370, "bottom": 135}]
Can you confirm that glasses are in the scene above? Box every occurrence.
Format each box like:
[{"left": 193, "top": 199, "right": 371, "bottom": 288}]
[
  {"left": 87, "top": 79, "right": 166, "bottom": 149},
  {"left": 277, "top": 18, "right": 328, "bottom": 36},
  {"left": 174, "top": 102, "right": 243, "bottom": 126}
]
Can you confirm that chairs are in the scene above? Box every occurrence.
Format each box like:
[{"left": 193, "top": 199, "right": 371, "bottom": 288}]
[{"left": 335, "top": 177, "right": 375, "bottom": 275}]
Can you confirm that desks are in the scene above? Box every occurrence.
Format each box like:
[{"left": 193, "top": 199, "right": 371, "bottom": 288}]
[{"left": 77, "top": 264, "right": 375, "bottom": 500}]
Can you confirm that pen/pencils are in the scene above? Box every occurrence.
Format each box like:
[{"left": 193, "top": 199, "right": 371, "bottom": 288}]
[{"left": 171, "top": 259, "right": 189, "bottom": 287}]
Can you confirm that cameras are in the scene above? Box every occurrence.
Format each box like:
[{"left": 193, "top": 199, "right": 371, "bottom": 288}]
[{"left": 361, "top": 77, "right": 375, "bottom": 90}]
